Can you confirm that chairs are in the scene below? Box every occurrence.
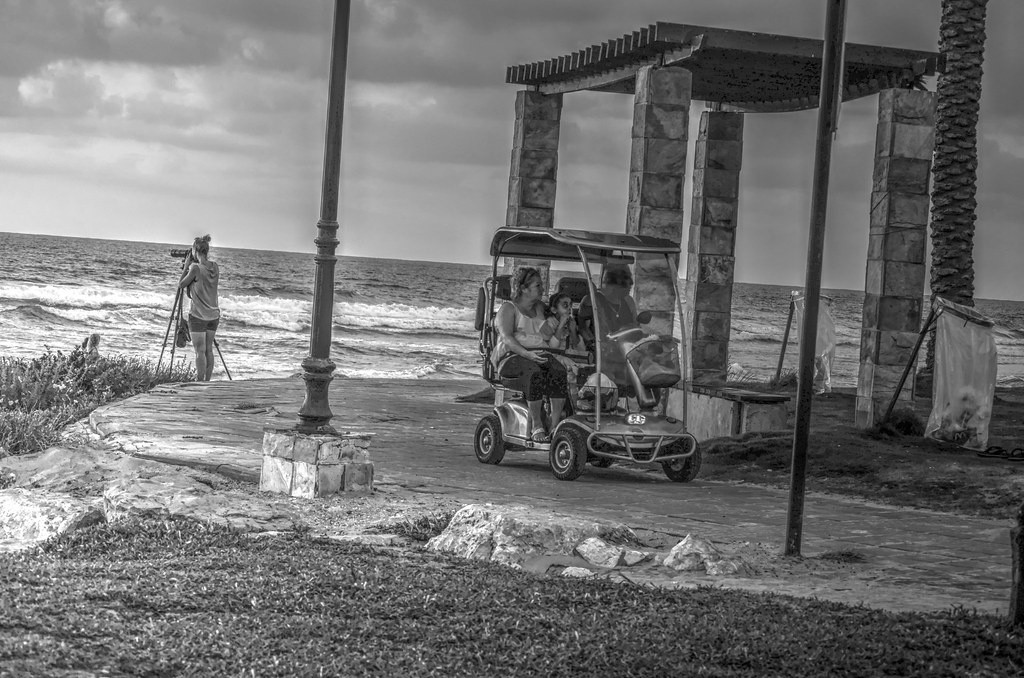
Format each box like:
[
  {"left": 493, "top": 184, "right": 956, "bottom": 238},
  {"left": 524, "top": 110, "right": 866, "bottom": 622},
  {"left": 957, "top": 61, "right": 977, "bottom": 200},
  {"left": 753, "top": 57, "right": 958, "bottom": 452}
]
[{"left": 482, "top": 276, "right": 597, "bottom": 388}]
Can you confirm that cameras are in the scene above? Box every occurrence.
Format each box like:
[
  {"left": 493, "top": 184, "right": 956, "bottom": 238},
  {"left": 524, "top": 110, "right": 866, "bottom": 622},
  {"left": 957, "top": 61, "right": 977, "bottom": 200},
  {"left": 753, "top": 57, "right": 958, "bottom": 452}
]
[{"left": 171, "top": 248, "right": 194, "bottom": 261}]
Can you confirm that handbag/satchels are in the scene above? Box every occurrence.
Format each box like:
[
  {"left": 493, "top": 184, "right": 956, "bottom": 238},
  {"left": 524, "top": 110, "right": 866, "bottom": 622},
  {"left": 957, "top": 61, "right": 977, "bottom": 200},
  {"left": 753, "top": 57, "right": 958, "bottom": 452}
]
[{"left": 176, "top": 319, "right": 192, "bottom": 348}]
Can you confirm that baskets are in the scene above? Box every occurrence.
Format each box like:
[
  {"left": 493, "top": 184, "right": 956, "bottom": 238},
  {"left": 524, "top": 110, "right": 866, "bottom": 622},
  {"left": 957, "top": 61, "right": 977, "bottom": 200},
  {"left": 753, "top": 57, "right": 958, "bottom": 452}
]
[
  {"left": 569, "top": 383, "right": 619, "bottom": 413},
  {"left": 625, "top": 339, "right": 680, "bottom": 388}
]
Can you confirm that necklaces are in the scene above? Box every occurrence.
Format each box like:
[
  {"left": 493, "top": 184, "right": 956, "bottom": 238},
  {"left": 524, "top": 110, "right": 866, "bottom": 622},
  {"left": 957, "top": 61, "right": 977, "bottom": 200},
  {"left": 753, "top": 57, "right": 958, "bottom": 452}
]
[{"left": 602, "top": 291, "right": 622, "bottom": 319}]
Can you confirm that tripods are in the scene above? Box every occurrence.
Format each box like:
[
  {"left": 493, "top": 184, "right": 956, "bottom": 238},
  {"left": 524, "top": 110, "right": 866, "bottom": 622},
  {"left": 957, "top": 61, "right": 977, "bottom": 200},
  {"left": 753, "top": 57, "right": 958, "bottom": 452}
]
[{"left": 154, "top": 285, "right": 232, "bottom": 381}]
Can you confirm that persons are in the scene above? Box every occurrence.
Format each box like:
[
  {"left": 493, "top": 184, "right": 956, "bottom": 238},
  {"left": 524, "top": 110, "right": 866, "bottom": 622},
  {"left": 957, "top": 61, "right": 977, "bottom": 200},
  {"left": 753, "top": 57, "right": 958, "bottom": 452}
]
[
  {"left": 178, "top": 235, "right": 219, "bottom": 382},
  {"left": 489, "top": 267, "right": 647, "bottom": 442}
]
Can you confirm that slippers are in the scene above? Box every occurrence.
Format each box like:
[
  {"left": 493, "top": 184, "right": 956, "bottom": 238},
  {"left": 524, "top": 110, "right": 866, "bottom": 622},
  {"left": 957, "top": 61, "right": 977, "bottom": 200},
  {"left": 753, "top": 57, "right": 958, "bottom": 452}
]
[
  {"left": 549, "top": 427, "right": 556, "bottom": 438},
  {"left": 532, "top": 429, "right": 550, "bottom": 442}
]
